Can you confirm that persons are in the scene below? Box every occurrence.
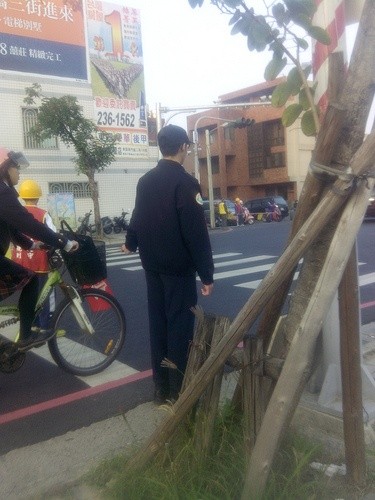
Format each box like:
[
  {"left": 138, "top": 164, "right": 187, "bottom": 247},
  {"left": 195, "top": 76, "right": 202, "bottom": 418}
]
[
  {"left": 234, "top": 198, "right": 246, "bottom": 226},
  {"left": 121, "top": 125, "right": 214, "bottom": 407},
  {"left": 5, "top": 179, "right": 57, "bottom": 332},
  {"left": 0, "top": 147, "right": 79, "bottom": 353},
  {"left": 294, "top": 199, "right": 299, "bottom": 212},
  {"left": 217, "top": 197, "right": 231, "bottom": 231},
  {"left": 262, "top": 198, "right": 276, "bottom": 223}
]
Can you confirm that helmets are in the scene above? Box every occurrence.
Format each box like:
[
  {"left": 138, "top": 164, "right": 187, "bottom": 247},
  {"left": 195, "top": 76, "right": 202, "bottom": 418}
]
[
  {"left": 0, "top": 147, "right": 22, "bottom": 170},
  {"left": 239, "top": 200, "right": 243, "bottom": 204},
  {"left": 235, "top": 198, "right": 240, "bottom": 201},
  {"left": 20, "top": 180, "right": 42, "bottom": 199},
  {"left": 275, "top": 205, "right": 279, "bottom": 208}
]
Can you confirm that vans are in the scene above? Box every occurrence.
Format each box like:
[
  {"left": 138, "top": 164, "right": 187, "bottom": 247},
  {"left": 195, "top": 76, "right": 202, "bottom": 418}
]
[
  {"left": 243, "top": 195, "right": 290, "bottom": 220},
  {"left": 204, "top": 199, "right": 237, "bottom": 225}
]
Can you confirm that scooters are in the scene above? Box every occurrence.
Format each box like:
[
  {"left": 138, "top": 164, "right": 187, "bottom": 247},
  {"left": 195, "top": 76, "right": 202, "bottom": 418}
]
[
  {"left": 238, "top": 205, "right": 255, "bottom": 225},
  {"left": 260, "top": 201, "right": 282, "bottom": 223},
  {"left": 112, "top": 208, "right": 130, "bottom": 233},
  {"left": 81, "top": 210, "right": 113, "bottom": 234}
]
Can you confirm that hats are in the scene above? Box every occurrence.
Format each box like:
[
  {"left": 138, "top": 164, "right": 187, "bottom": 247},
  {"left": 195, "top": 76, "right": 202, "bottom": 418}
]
[{"left": 158, "top": 124, "right": 196, "bottom": 146}]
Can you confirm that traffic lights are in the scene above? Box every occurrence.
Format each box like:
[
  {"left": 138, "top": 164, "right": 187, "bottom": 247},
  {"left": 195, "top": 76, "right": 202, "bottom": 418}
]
[{"left": 234, "top": 116, "right": 256, "bottom": 127}]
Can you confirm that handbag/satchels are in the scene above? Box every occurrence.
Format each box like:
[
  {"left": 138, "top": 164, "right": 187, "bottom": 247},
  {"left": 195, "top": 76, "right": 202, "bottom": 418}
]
[
  {"left": 82, "top": 278, "right": 114, "bottom": 313},
  {"left": 59, "top": 220, "right": 103, "bottom": 273}
]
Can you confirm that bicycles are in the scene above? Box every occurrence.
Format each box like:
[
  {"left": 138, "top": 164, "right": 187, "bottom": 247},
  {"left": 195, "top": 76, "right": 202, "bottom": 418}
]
[{"left": 0, "top": 238, "right": 127, "bottom": 375}]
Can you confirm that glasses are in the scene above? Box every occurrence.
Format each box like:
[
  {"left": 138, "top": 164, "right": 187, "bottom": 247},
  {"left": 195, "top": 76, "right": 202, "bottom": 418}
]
[{"left": 186, "top": 143, "right": 190, "bottom": 148}]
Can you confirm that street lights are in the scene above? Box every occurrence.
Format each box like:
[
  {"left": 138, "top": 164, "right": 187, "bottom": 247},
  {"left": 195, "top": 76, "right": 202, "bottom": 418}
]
[{"left": 204, "top": 121, "right": 229, "bottom": 229}]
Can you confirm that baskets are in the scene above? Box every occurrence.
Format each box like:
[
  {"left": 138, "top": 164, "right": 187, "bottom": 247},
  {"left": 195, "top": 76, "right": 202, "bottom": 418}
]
[
  {"left": 60, "top": 240, "right": 107, "bottom": 283},
  {"left": 78, "top": 217, "right": 84, "bottom": 222},
  {"left": 111, "top": 220, "right": 118, "bottom": 227}
]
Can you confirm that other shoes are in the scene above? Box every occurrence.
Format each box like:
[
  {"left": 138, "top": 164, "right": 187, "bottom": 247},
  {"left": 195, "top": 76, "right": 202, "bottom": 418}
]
[{"left": 153, "top": 389, "right": 179, "bottom": 404}]
